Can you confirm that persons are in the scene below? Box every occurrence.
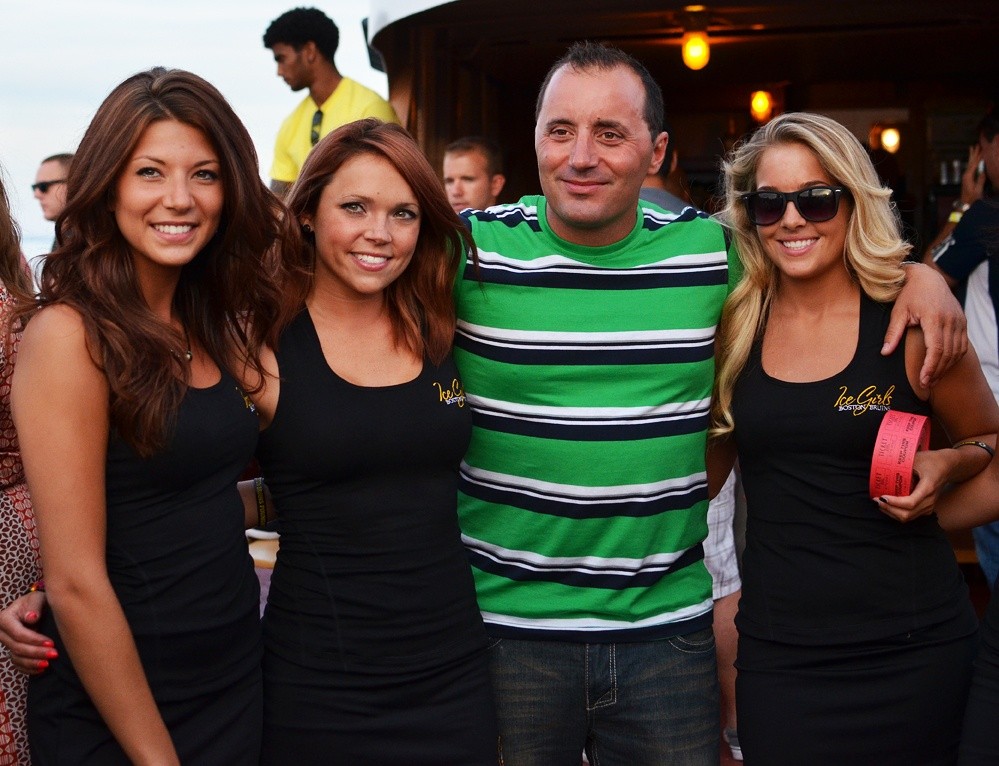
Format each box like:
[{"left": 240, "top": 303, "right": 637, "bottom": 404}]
[{"left": 0, "top": 7, "right": 999, "bottom": 766}]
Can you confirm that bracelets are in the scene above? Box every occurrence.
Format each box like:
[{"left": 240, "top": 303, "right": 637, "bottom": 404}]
[
  {"left": 24, "top": 581, "right": 45, "bottom": 594},
  {"left": 952, "top": 199, "right": 970, "bottom": 209},
  {"left": 948, "top": 212, "right": 963, "bottom": 223},
  {"left": 952, "top": 440, "right": 995, "bottom": 456},
  {"left": 900, "top": 260, "right": 921, "bottom": 267},
  {"left": 253, "top": 478, "right": 267, "bottom": 527}
]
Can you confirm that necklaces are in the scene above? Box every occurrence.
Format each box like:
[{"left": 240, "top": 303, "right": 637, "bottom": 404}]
[{"left": 170, "top": 320, "right": 193, "bottom": 364}]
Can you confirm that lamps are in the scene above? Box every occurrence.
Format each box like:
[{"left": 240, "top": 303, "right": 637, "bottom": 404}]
[{"left": 679, "top": 0, "right": 710, "bottom": 70}]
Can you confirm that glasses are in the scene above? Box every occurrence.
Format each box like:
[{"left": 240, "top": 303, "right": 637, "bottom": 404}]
[
  {"left": 742, "top": 186, "right": 845, "bottom": 226},
  {"left": 310, "top": 110, "right": 323, "bottom": 146},
  {"left": 32, "top": 180, "right": 66, "bottom": 194}
]
